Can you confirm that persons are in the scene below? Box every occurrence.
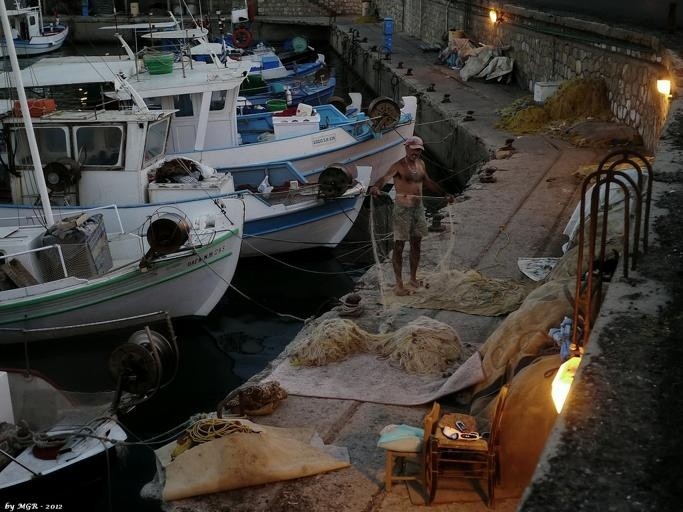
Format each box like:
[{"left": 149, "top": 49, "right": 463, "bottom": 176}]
[{"left": 370, "top": 136, "right": 455, "bottom": 296}]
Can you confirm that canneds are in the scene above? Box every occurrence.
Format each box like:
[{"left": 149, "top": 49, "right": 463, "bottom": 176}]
[{"left": 232, "top": 29, "right": 251, "bottom": 47}]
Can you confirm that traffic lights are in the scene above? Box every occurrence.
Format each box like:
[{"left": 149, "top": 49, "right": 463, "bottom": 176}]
[{"left": 377, "top": 423, "right": 423, "bottom": 452}]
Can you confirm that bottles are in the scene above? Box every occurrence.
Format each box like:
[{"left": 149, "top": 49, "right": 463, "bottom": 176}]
[{"left": 403, "top": 136, "right": 425, "bottom": 151}]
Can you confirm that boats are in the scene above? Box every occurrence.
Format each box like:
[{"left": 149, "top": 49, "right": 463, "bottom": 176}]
[{"left": 1, "top": 0, "right": 418, "bottom": 491}]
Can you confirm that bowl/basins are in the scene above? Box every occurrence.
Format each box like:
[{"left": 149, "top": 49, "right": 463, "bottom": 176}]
[{"left": 404, "top": 157, "right": 418, "bottom": 174}]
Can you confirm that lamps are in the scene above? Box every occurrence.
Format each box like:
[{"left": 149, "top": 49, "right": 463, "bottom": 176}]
[{"left": 384, "top": 385, "right": 509, "bottom": 509}]
